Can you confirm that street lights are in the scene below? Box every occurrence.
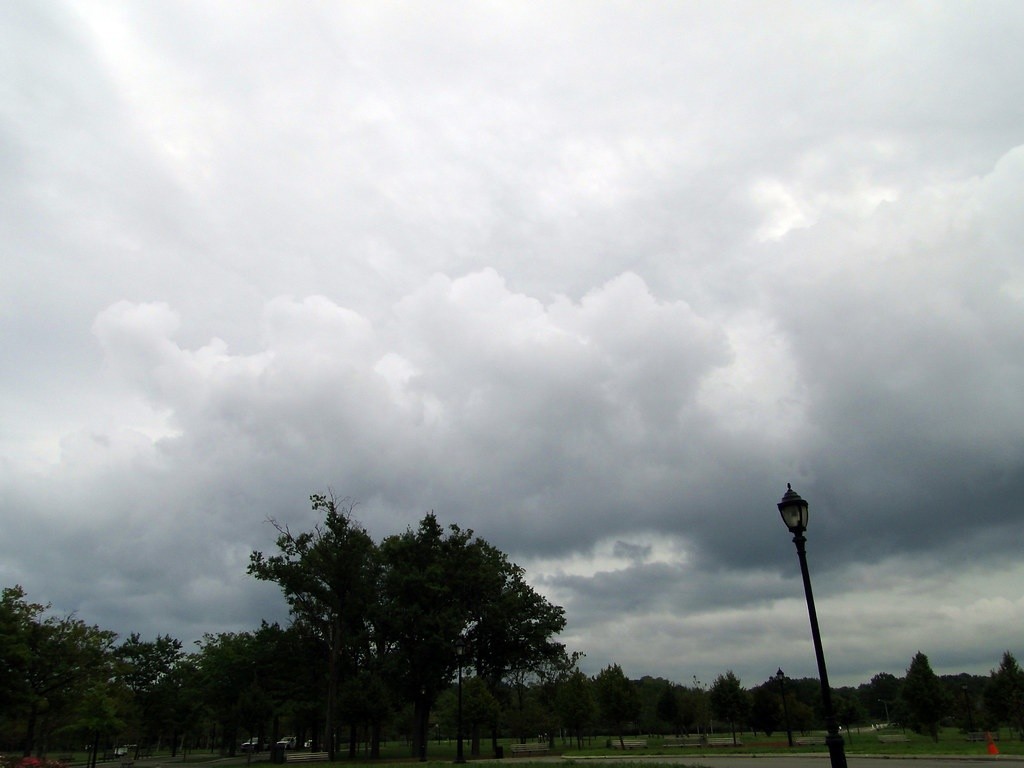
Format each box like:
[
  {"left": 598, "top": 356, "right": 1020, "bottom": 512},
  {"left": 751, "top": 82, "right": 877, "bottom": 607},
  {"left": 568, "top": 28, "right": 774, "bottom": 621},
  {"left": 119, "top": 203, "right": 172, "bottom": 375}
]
[
  {"left": 776, "top": 666, "right": 793, "bottom": 746},
  {"left": 777, "top": 482, "right": 848, "bottom": 768}
]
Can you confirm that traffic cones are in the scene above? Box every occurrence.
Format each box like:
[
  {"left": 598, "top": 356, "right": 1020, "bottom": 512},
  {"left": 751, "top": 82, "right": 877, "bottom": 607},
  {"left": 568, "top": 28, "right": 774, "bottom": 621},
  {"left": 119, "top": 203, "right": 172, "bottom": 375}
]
[{"left": 986, "top": 730, "right": 1000, "bottom": 756}]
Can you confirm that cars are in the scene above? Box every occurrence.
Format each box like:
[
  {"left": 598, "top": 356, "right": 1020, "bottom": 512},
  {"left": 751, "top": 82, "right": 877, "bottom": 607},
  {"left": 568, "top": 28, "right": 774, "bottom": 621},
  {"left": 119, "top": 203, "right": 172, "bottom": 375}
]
[
  {"left": 277, "top": 736, "right": 297, "bottom": 751},
  {"left": 241, "top": 737, "right": 271, "bottom": 752}
]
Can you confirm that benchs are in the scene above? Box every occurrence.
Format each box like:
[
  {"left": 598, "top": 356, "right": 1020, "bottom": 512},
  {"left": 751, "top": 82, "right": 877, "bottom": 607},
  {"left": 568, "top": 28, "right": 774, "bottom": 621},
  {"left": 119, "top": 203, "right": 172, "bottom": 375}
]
[
  {"left": 611, "top": 740, "right": 648, "bottom": 750},
  {"left": 661, "top": 739, "right": 702, "bottom": 748},
  {"left": 876, "top": 734, "right": 912, "bottom": 744},
  {"left": 965, "top": 732, "right": 1000, "bottom": 744},
  {"left": 795, "top": 737, "right": 829, "bottom": 747},
  {"left": 286, "top": 752, "right": 330, "bottom": 765},
  {"left": 707, "top": 737, "right": 743, "bottom": 748},
  {"left": 510, "top": 743, "right": 550, "bottom": 758},
  {"left": 57, "top": 754, "right": 75, "bottom": 763}
]
